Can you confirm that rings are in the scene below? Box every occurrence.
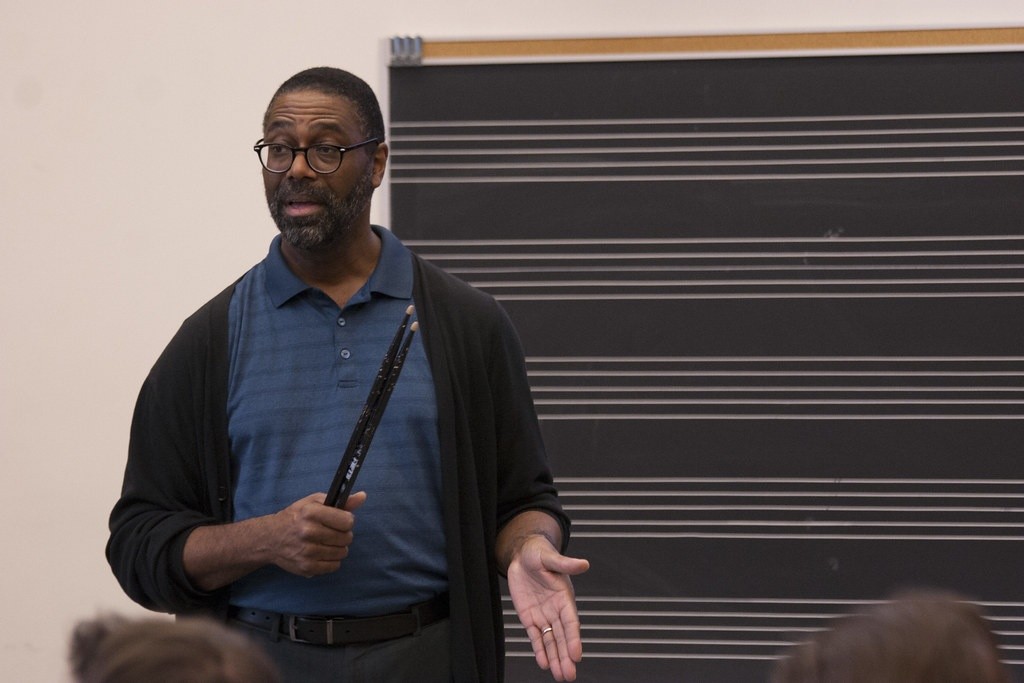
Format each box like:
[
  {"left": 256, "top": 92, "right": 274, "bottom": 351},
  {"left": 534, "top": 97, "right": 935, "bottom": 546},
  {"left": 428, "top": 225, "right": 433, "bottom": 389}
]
[{"left": 541, "top": 627, "right": 552, "bottom": 637}]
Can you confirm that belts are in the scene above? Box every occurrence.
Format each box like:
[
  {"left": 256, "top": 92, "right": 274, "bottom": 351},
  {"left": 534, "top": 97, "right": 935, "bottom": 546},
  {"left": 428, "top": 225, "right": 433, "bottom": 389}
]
[{"left": 230, "top": 606, "right": 445, "bottom": 646}]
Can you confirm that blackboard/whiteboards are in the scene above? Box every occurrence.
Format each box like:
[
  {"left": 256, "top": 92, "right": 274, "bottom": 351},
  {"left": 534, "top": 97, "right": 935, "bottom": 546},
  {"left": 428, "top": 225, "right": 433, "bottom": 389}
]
[{"left": 376, "top": 33, "right": 1024, "bottom": 682}]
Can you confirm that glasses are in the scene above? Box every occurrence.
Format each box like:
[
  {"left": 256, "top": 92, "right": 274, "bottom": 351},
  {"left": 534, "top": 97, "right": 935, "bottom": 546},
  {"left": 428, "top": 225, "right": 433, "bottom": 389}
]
[{"left": 253, "top": 136, "right": 385, "bottom": 173}]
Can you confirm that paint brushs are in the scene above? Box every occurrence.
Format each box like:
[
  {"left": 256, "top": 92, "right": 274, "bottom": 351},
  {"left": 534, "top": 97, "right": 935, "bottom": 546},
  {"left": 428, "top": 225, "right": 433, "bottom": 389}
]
[{"left": 321, "top": 302, "right": 421, "bottom": 518}]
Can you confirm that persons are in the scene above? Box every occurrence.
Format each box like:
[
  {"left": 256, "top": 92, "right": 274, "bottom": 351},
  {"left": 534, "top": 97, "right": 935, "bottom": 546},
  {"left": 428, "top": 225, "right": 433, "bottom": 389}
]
[
  {"left": 105, "top": 66, "right": 588, "bottom": 683},
  {"left": 777, "top": 590, "right": 1008, "bottom": 683},
  {"left": 68, "top": 613, "right": 280, "bottom": 683}
]
